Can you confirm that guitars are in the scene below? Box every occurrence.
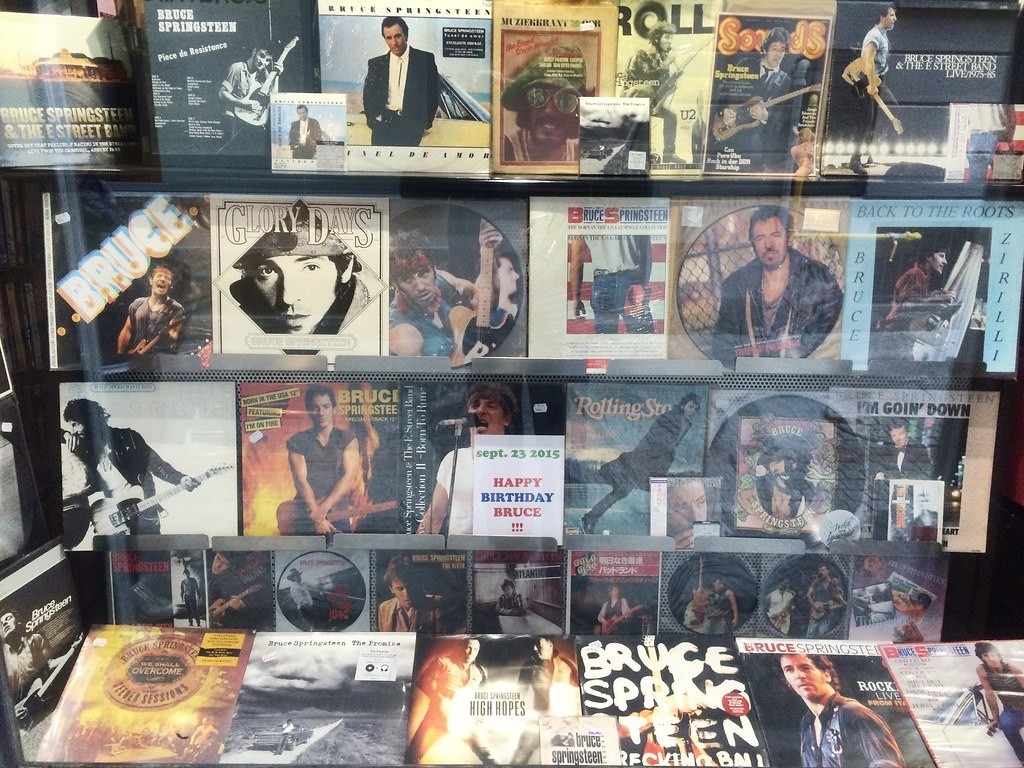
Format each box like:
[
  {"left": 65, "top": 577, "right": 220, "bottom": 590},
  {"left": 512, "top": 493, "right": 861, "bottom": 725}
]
[
  {"left": 841, "top": 57, "right": 905, "bottom": 135},
  {"left": 89, "top": 461, "right": 237, "bottom": 537},
  {"left": 234, "top": 34, "right": 301, "bottom": 127},
  {"left": 209, "top": 581, "right": 265, "bottom": 622},
  {"left": 449, "top": 218, "right": 513, "bottom": 366},
  {"left": 809, "top": 599, "right": 846, "bottom": 620},
  {"left": 595, "top": 603, "right": 645, "bottom": 636},
  {"left": 275, "top": 497, "right": 399, "bottom": 536},
  {"left": 14, "top": 632, "right": 86, "bottom": 730},
  {"left": 712, "top": 82, "right": 822, "bottom": 141},
  {"left": 127, "top": 317, "right": 190, "bottom": 354},
  {"left": 910, "top": 236, "right": 983, "bottom": 348}
]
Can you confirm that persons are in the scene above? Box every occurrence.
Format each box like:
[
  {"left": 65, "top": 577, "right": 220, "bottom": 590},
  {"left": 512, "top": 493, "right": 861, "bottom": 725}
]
[{"left": 0, "top": 0, "right": 1024, "bottom": 768}]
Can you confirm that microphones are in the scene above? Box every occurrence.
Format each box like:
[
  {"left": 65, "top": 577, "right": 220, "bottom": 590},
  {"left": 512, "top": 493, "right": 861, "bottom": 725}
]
[{"left": 668, "top": 48, "right": 680, "bottom": 71}]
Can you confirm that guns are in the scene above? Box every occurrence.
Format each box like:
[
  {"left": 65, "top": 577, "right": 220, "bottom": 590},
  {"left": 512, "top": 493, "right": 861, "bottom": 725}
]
[{"left": 648, "top": 36, "right": 713, "bottom": 115}]
